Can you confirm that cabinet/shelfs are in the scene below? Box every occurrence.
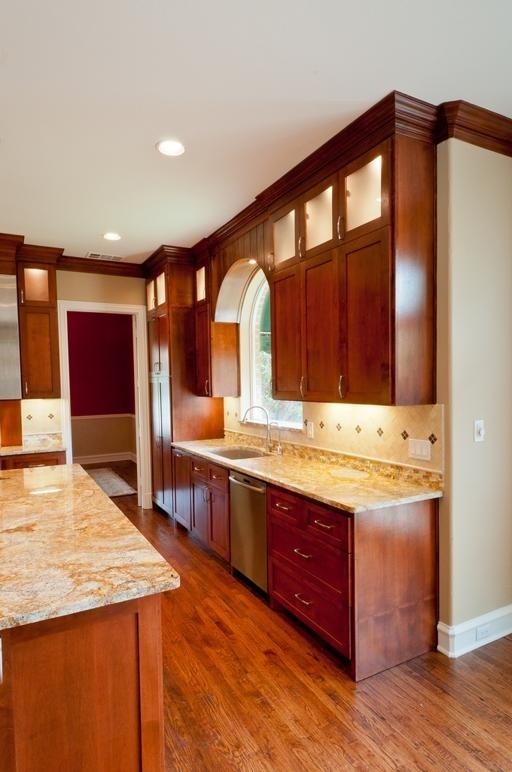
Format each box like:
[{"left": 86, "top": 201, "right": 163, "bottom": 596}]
[
  {"left": 16, "top": 261, "right": 62, "bottom": 398},
  {"left": 144, "top": 262, "right": 224, "bottom": 530},
  {"left": 190, "top": 456, "right": 229, "bottom": 560},
  {"left": 172, "top": 449, "right": 189, "bottom": 530},
  {"left": 268, "top": 486, "right": 439, "bottom": 683},
  {"left": 195, "top": 258, "right": 211, "bottom": 394}
]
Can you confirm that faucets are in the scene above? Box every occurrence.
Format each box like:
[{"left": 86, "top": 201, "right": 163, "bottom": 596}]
[
  {"left": 242, "top": 406, "right": 273, "bottom": 455},
  {"left": 269, "top": 422, "right": 284, "bottom": 456}
]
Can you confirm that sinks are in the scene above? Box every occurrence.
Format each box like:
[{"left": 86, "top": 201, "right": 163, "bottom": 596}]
[{"left": 207, "top": 445, "right": 277, "bottom": 461}]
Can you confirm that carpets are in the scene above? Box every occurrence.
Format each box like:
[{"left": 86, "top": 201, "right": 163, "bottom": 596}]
[{"left": 85, "top": 467, "right": 138, "bottom": 497}]
